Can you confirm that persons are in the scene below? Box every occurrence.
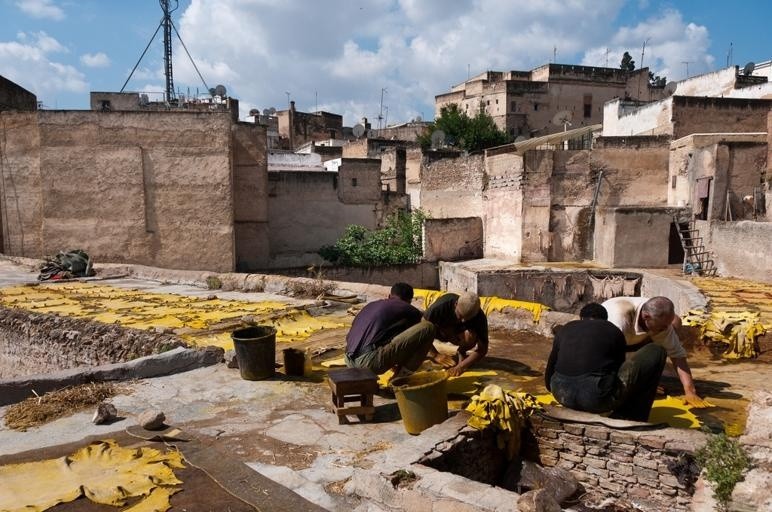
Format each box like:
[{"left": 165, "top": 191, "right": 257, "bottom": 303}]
[
  {"left": 544, "top": 296, "right": 711, "bottom": 421},
  {"left": 344, "top": 283, "right": 435, "bottom": 387},
  {"left": 421, "top": 291, "right": 488, "bottom": 377}
]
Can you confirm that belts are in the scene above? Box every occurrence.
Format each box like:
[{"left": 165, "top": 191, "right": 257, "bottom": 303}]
[{"left": 347, "top": 345, "right": 375, "bottom": 360}]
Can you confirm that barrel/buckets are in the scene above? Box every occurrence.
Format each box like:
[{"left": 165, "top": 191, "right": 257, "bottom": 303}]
[
  {"left": 231, "top": 325, "right": 278, "bottom": 381},
  {"left": 389, "top": 370, "right": 452, "bottom": 433}
]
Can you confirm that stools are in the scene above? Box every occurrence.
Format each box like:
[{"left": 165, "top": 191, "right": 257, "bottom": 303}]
[{"left": 328, "top": 367, "right": 380, "bottom": 426}]
